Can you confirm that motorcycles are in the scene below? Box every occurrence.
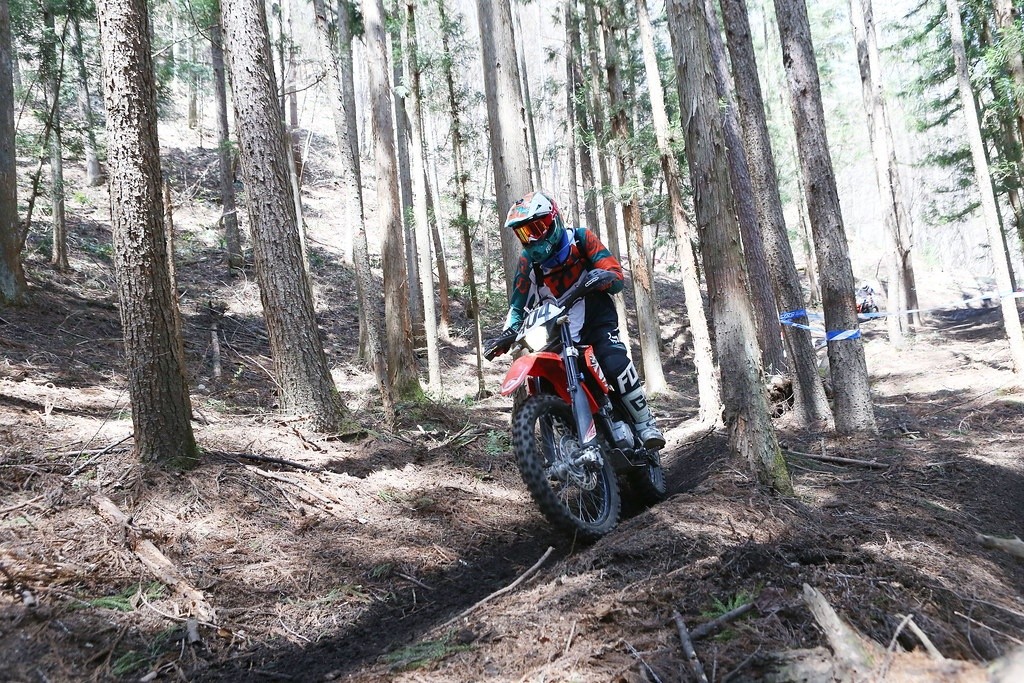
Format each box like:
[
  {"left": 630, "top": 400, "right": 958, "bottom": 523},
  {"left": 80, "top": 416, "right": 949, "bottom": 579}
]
[
  {"left": 856, "top": 297, "right": 879, "bottom": 313},
  {"left": 482, "top": 275, "right": 665, "bottom": 543}
]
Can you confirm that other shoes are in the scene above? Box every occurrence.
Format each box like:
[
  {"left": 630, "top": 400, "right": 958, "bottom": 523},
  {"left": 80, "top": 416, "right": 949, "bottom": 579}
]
[{"left": 640, "top": 425, "right": 666, "bottom": 449}]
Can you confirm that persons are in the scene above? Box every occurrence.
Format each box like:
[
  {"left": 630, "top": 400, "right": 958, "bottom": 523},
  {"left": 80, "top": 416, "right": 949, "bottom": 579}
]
[{"left": 492, "top": 191, "right": 667, "bottom": 452}]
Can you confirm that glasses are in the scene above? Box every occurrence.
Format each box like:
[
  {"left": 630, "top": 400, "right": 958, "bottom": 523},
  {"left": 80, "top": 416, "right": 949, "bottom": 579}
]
[{"left": 512, "top": 210, "right": 558, "bottom": 245}]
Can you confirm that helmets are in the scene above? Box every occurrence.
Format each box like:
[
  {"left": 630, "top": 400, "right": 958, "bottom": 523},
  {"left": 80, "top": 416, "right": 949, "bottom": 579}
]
[{"left": 504, "top": 191, "right": 562, "bottom": 261}]
